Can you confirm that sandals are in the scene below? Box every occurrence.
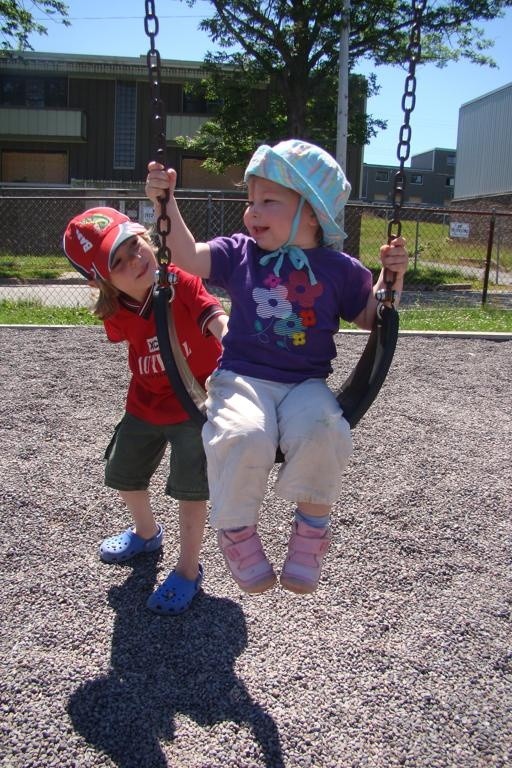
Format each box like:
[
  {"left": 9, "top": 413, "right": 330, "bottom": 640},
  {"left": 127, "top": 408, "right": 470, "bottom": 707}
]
[
  {"left": 145, "top": 560, "right": 203, "bottom": 616},
  {"left": 100, "top": 521, "right": 163, "bottom": 564}
]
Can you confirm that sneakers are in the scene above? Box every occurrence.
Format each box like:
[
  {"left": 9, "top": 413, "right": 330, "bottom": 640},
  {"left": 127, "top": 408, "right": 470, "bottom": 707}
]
[
  {"left": 218, "top": 524, "right": 277, "bottom": 593},
  {"left": 280, "top": 520, "right": 332, "bottom": 595}
]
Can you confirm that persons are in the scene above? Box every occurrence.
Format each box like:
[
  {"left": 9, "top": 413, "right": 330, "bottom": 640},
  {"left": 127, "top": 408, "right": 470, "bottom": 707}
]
[
  {"left": 141, "top": 136, "right": 409, "bottom": 594},
  {"left": 58, "top": 197, "right": 239, "bottom": 615}
]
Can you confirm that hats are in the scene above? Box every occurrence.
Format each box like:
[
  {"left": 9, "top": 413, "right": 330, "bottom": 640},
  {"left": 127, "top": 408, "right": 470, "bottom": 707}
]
[
  {"left": 243, "top": 137, "right": 353, "bottom": 247},
  {"left": 62, "top": 206, "right": 146, "bottom": 284}
]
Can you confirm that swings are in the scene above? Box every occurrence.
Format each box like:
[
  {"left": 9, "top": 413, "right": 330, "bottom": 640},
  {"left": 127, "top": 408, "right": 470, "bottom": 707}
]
[{"left": 145, "top": 1, "right": 426, "bottom": 464}]
